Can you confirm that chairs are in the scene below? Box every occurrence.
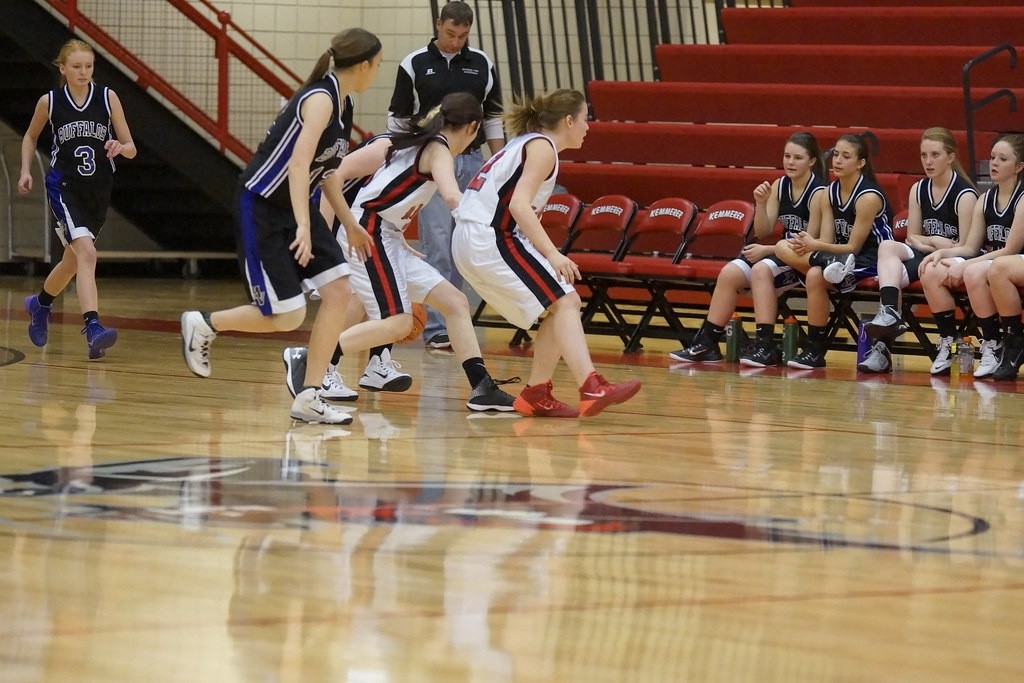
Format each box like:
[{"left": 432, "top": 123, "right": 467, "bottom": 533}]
[{"left": 462, "top": 197, "right": 1023, "bottom": 375}]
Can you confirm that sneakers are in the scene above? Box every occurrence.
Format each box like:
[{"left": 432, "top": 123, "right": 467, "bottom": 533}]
[
  {"left": 993, "top": 332, "right": 1024, "bottom": 381},
  {"left": 24, "top": 295, "right": 53, "bottom": 346},
  {"left": 973, "top": 336, "right": 1007, "bottom": 378},
  {"left": 669, "top": 343, "right": 724, "bottom": 363},
  {"left": 822, "top": 253, "right": 855, "bottom": 284},
  {"left": 858, "top": 340, "right": 894, "bottom": 374},
  {"left": 289, "top": 386, "right": 353, "bottom": 425},
  {"left": 465, "top": 374, "right": 521, "bottom": 412},
  {"left": 80, "top": 319, "right": 118, "bottom": 359},
  {"left": 786, "top": 345, "right": 826, "bottom": 369},
  {"left": 930, "top": 335, "right": 964, "bottom": 375},
  {"left": 282, "top": 345, "right": 308, "bottom": 398},
  {"left": 512, "top": 380, "right": 580, "bottom": 418},
  {"left": 318, "top": 361, "right": 359, "bottom": 401},
  {"left": 359, "top": 348, "right": 413, "bottom": 392},
  {"left": 179, "top": 310, "right": 218, "bottom": 378},
  {"left": 863, "top": 302, "right": 908, "bottom": 339},
  {"left": 578, "top": 371, "right": 642, "bottom": 417},
  {"left": 740, "top": 335, "right": 783, "bottom": 367}
]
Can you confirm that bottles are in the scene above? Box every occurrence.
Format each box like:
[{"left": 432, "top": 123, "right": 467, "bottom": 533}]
[
  {"left": 782, "top": 315, "right": 799, "bottom": 365},
  {"left": 958, "top": 337, "right": 975, "bottom": 376},
  {"left": 725, "top": 312, "right": 743, "bottom": 361},
  {"left": 856, "top": 319, "right": 873, "bottom": 369},
  {"left": 950, "top": 333, "right": 965, "bottom": 377}
]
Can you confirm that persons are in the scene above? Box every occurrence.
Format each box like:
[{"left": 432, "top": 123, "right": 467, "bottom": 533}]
[
  {"left": 670, "top": 126, "right": 1024, "bottom": 381},
  {"left": 17, "top": 38, "right": 137, "bottom": 359},
  {"left": 180, "top": 1, "right": 641, "bottom": 425}
]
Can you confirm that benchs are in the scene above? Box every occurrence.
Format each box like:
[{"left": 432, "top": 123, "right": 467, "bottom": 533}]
[{"left": 551, "top": 0, "right": 1024, "bottom": 216}]
